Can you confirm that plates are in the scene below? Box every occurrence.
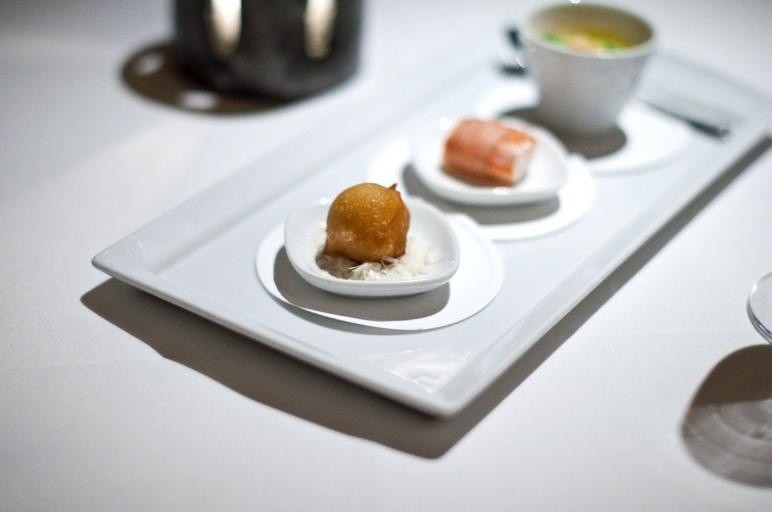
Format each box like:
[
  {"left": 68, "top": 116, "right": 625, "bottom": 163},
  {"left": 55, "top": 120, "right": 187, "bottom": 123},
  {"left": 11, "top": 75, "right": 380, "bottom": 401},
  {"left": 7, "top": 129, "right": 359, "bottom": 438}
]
[
  {"left": 414, "top": 122, "right": 568, "bottom": 205},
  {"left": 285, "top": 197, "right": 460, "bottom": 299},
  {"left": 90, "top": 21, "right": 772, "bottom": 416}
]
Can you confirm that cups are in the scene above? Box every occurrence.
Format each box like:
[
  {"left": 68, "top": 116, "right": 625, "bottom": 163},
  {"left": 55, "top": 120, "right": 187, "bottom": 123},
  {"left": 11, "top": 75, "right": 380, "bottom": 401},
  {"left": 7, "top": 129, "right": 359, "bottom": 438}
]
[
  {"left": 168, "top": 1, "right": 363, "bottom": 99},
  {"left": 506, "top": 1, "right": 655, "bottom": 141}
]
